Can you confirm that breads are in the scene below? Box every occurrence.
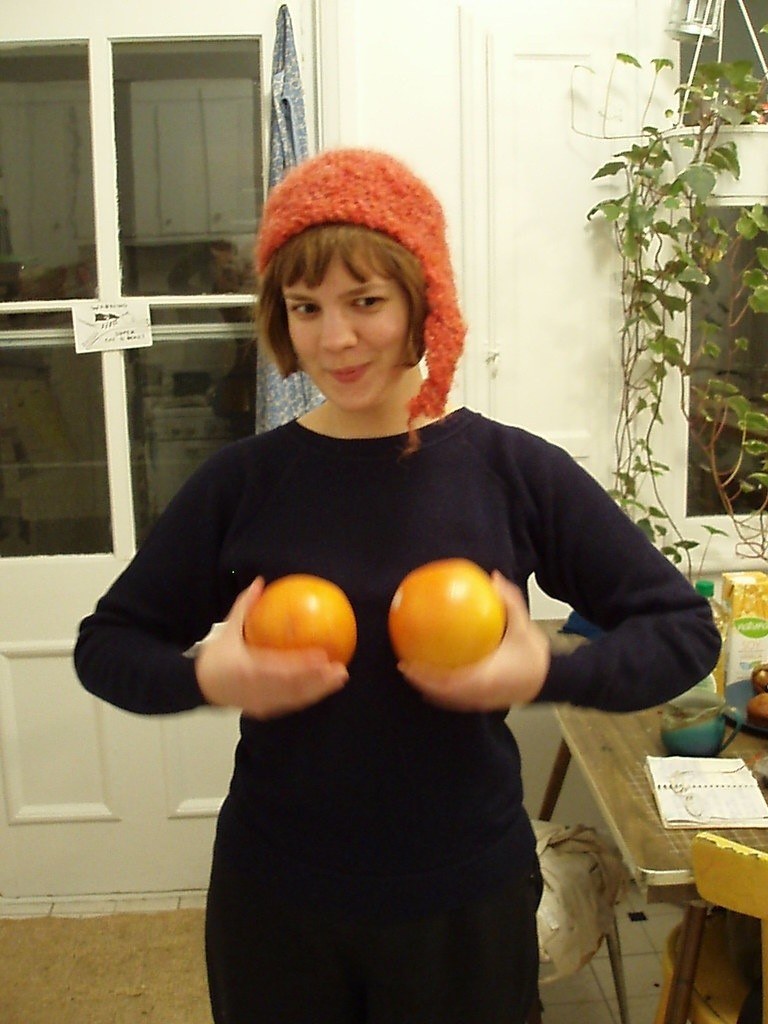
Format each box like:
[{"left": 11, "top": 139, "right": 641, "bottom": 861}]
[{"left": 746, "top": 663, "right": 768, "bottom": 730}]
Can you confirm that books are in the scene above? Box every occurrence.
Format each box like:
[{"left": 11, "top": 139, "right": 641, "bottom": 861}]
[{"left": 643, "top": 756, "right": 768, "bottom": 830}]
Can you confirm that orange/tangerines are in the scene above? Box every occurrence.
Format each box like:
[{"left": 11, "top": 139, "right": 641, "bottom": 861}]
[
  {"left": 243, "top": 573, "right": 356, "bottom": 670},
  {"left": 389, "top": 557, "right": 508, "bottom": 668}
]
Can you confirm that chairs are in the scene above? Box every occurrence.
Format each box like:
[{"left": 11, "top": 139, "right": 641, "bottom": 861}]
[
  {"left": 656, "top": 832, "right": 768, "bottom": 1024},
  {"left": 530, "top": 820, "right": 631, "bottom": 1024}
]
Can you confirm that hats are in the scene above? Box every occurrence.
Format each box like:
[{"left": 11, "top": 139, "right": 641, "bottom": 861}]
[{"left": 262, "top": 147, "right": 466, "bottom": 456}]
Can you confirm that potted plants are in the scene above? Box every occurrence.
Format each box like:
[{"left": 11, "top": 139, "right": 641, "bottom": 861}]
[{"left": 587, "top": 0, "right": 768, "bottom": 597}]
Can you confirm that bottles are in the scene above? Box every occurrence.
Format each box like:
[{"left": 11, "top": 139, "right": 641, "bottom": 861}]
[{"left": 691, "top": 581, "right": 727, "bottom": 689}]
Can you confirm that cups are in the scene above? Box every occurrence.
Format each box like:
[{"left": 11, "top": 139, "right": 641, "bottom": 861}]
[{"left": 662, "top": 690, "right": 744, "bottom": 756}]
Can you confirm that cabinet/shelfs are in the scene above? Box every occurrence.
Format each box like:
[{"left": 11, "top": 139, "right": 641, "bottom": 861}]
[{"left": 0, "top": 79, "right": 258, "bottom": 278}]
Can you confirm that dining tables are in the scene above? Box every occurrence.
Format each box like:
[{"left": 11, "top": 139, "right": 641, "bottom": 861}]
[{"left": 521, "top": 619, "right": 768, "bottom": 1024}]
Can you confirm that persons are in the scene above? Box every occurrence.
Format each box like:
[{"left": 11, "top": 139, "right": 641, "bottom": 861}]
[
  {"left": 199, "top": 239, "right": 260, "bottom": 436},
  {"left": 74, "top": 148, "right": 721, "bottom": 1024}
]
